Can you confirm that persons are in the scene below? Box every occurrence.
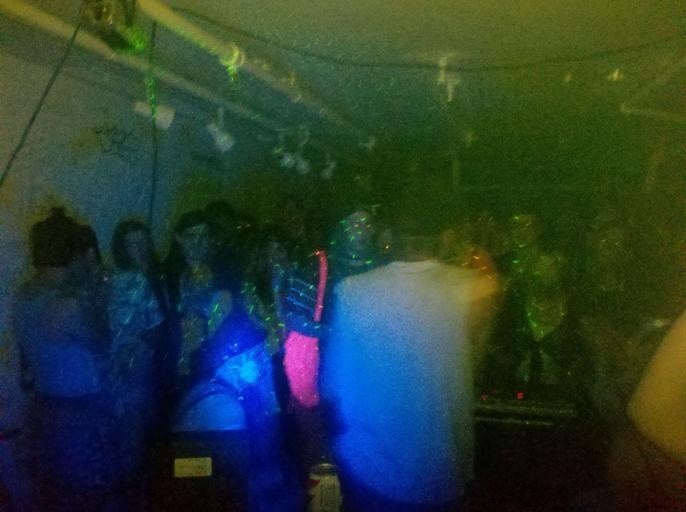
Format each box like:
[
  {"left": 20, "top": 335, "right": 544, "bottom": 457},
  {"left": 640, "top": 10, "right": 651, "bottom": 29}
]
[{"left": 0, "top": 179, "right": 685, "bottom": 512}]
